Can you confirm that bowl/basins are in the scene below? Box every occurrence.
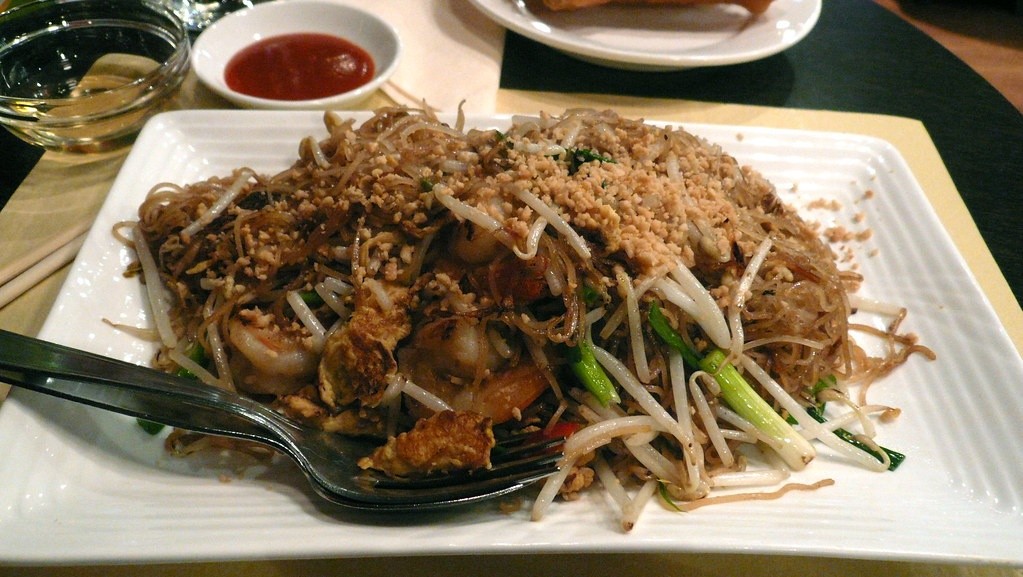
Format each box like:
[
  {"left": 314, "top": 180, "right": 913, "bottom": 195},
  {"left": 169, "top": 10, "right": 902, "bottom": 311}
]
[
  {"left": 0, "top": 0, "right": 190, "bottom": 161},
  {"left": 191, "top": 0, "right": 403, "bottom": 110}
]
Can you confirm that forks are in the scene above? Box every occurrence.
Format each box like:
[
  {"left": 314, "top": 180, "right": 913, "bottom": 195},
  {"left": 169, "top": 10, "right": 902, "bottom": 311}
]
[{"left": 0, "top": 328, "right": 567, "bottom": 509}]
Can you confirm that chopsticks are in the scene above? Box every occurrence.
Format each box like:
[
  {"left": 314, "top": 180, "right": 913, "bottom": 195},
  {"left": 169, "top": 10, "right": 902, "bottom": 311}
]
[{"left": 0, "top": 220, "right": 95, "bottom": 308}]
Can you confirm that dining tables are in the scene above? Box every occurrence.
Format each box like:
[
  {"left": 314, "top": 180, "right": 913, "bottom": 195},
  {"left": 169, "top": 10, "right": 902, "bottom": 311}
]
[{"left": 0, "top": 0, "right": 1023, "bottom": 577}]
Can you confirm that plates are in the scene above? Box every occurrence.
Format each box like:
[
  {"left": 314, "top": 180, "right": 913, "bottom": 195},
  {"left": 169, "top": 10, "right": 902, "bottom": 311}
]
[
  {"left": 0, "top": 110, "right": 1023, "bottom": 566},
  {"left": 468, "top": 0, "right": 823, "bottom": 72}
]
